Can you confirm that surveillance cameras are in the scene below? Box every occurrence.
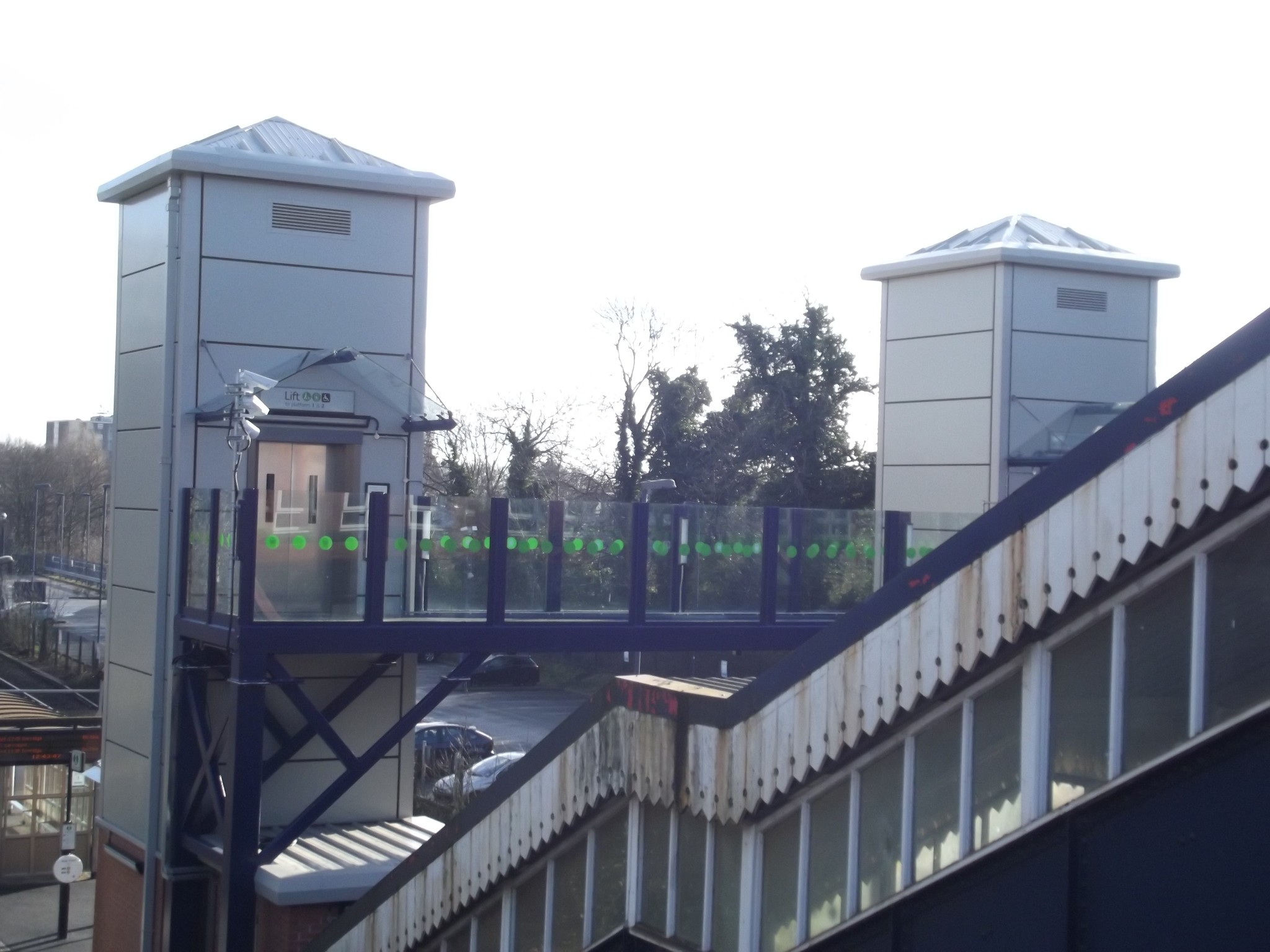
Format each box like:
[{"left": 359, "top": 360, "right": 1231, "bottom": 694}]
[{"left": 236, "top": 369, "right": 278, "bottom": 440}]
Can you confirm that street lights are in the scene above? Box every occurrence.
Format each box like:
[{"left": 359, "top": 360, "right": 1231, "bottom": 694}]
[
  {"left": 55, "top": 492, "right": 65, "bottom": 578},
  {"left": 97, "top": 483, "right": 111, "bottom": 643},
  {"left": 82, "top": 493, "right": 91, "bottom": 577},
  {"left": 628, "top": 476, "right": 678, "bottom": 676},
  {"left": 27, "top": 483, "right": 52, "bottom": 658}
]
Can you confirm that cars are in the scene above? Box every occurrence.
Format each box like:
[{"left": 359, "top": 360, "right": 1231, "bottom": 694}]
[
  {"left": 432, "top": 751, "right": 528, "bottom": 805},
  {"left": 467, "top": 653, "right": 540, "bottom": 688},
  {"left": 413, "top": 720, "right": 495, "bottom": 763}
]
